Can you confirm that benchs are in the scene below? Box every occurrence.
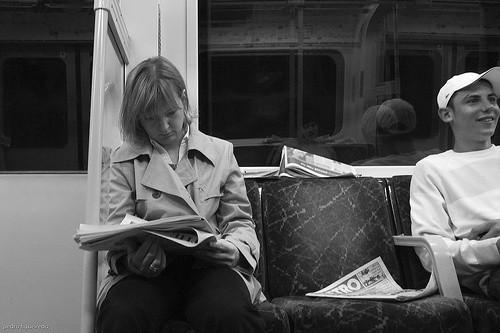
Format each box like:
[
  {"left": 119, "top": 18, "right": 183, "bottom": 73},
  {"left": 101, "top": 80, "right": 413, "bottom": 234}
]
[{"left": 244, "top": 173, "right": 500, "bottom": 333}]
